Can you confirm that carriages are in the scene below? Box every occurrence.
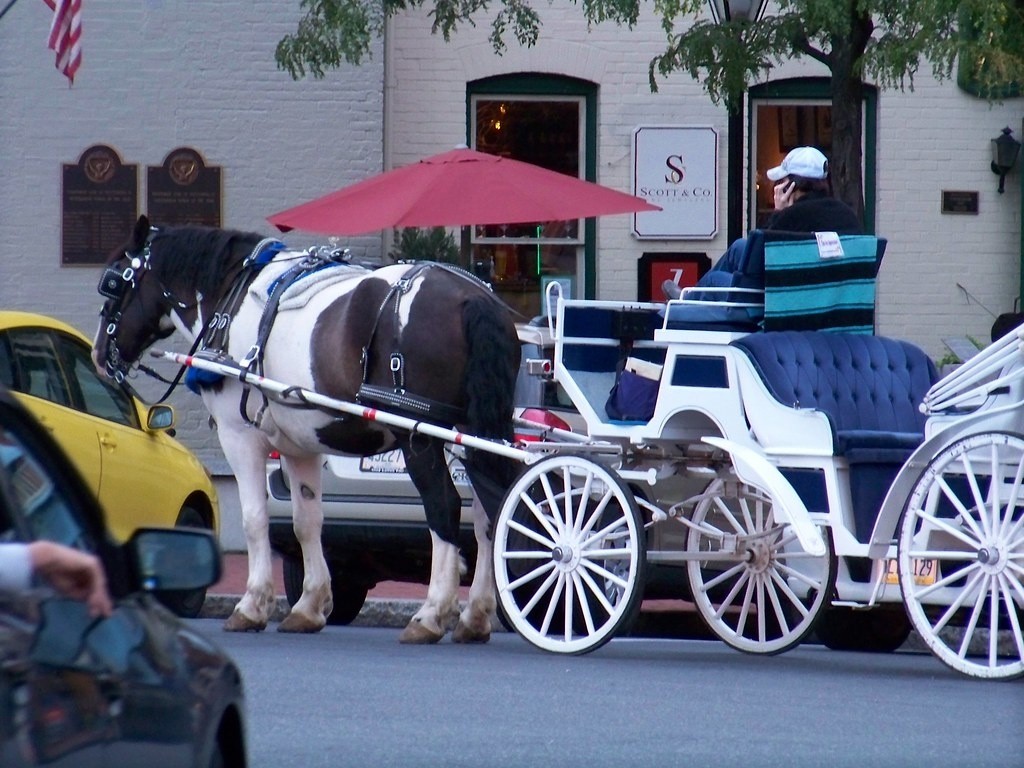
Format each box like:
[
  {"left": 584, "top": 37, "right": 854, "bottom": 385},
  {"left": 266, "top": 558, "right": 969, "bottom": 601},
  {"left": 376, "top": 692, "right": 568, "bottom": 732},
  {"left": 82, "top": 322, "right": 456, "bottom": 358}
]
[{"left": 89, "top": 214, "right": 1023, "bottom": 683}]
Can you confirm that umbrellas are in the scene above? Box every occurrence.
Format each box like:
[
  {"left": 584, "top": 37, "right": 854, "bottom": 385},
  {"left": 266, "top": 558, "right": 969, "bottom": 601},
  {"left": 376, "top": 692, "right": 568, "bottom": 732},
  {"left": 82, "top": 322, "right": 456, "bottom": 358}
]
[{"left": 266, "top": 145, "right": 664, "bottom": 274}]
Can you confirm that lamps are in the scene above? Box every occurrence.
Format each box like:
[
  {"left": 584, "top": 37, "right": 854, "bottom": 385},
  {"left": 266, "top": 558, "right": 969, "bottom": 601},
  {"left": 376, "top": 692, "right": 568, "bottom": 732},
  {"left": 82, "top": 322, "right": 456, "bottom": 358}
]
[{"left": 990, "top": 125, "right": 1022, "bottom": 193}]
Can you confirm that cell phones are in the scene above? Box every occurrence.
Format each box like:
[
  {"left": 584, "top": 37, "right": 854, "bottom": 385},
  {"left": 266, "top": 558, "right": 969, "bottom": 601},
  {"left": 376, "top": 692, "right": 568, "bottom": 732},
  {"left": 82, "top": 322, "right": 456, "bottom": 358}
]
[{"left": 783, "top": 178, "right": 801, "bottom": 193}]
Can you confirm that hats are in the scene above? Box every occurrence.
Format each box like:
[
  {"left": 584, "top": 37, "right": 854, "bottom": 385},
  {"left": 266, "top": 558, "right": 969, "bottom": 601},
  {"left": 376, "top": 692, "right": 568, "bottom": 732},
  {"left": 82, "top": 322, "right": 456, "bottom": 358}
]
[{"left": 767, "top": 146, "right": 828, "bottom": 182}]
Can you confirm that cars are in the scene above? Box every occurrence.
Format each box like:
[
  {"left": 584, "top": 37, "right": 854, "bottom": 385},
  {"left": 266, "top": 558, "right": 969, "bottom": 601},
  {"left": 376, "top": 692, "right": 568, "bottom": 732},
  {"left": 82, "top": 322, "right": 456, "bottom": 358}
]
[
  {"left": 1, "top": 310, "right": 222, "bottom": 618},
  {"left": 0, "top": 388, "right": 248, "bottom": 767}
]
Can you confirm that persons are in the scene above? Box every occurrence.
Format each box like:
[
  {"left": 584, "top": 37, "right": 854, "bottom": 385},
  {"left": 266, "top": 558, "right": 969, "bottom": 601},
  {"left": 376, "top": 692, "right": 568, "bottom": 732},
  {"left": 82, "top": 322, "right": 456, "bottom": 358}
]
[
  {"left": 660, "top": 147, "right": 863, "bottom": 305},
  {"left": 1, "top": 539, "right": 113, "bottom": 619}
]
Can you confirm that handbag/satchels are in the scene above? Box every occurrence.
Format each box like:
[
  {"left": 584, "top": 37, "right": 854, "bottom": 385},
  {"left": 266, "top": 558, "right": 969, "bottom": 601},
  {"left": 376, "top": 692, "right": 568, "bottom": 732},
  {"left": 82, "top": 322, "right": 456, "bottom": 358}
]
[{"left": 605, "top": 337, "right": 664, "bottom": 421}]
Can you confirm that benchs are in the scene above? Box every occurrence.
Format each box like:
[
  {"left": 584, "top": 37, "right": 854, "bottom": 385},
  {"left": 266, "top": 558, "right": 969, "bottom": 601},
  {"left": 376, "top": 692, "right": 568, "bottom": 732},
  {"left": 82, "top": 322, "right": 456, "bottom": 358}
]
[
  {"left": 653, "top": 228, "right": 888, "bottom": 342},
  {"left": 727, "top": 322, "right": 1024, "bottom": 547}
]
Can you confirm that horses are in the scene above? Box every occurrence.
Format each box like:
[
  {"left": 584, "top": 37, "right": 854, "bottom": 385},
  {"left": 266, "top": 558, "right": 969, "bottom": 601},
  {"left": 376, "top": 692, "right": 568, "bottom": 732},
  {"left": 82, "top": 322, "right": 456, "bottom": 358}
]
[{"left": 91, "top": 215, "right": 523, "bottom": 645}]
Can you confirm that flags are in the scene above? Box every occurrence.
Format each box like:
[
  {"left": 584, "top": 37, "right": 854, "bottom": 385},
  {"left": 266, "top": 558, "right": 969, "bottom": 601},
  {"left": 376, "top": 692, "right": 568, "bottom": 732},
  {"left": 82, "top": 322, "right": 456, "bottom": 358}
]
[{"left": 42, "top": 0, "right": 83, "bottom": 88}]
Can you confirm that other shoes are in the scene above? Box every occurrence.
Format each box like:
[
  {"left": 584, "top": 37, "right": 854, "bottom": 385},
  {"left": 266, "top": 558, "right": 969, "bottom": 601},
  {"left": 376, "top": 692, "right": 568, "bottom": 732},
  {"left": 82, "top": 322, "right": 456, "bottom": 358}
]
[{"left": 661, "top": 279, "right": 682, "bottom": 300}]
